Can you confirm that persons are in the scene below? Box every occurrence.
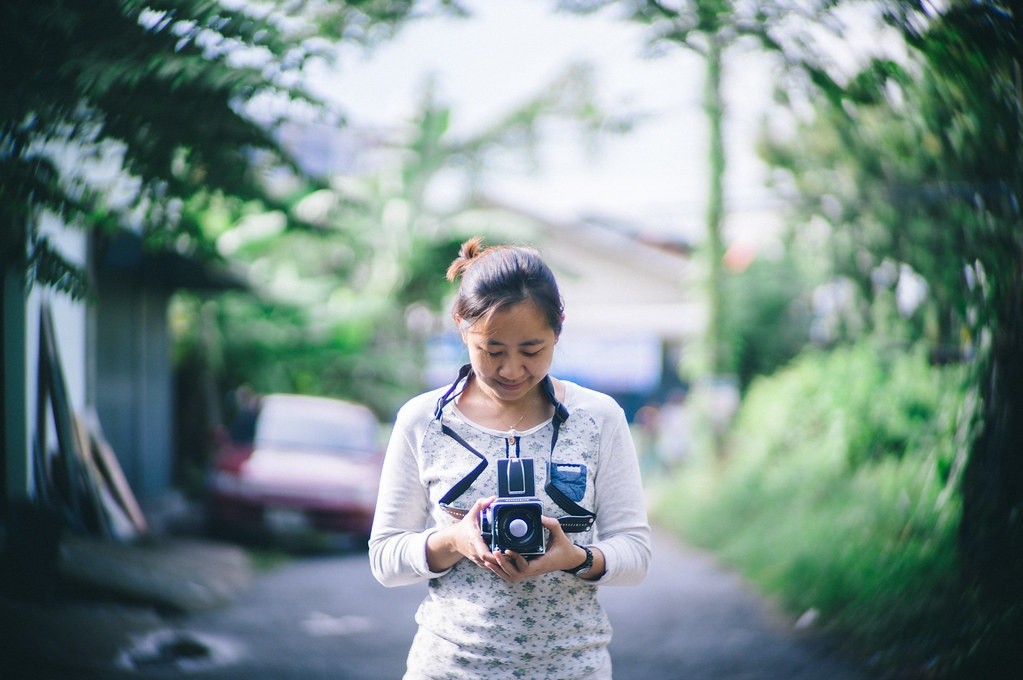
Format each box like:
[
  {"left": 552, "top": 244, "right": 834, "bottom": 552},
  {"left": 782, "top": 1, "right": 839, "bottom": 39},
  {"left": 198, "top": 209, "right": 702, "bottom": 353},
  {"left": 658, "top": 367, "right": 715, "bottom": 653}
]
[{"left": 369, "top": 238, "right": 648, "bottom": 680}]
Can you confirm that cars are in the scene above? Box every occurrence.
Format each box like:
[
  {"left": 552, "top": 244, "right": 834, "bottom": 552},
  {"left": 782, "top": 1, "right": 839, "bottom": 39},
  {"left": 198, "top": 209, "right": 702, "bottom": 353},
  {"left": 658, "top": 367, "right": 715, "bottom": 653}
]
[{"left": 207, "top": 391, "right": 392, "bottom": 550}]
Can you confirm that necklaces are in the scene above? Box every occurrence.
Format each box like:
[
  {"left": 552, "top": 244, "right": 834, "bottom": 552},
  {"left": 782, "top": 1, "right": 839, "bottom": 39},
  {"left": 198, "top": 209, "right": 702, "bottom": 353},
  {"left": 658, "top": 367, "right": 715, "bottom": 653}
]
[{"left": 479, "top": 388, "right": 538, "bottom": 444}]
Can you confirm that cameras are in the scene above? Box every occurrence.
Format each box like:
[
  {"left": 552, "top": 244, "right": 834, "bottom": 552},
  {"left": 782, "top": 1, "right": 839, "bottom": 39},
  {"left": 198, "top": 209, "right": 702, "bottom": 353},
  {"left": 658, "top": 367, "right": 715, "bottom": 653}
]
[{"left": 480, "top": 458, "right": 545, "bottom": 556}]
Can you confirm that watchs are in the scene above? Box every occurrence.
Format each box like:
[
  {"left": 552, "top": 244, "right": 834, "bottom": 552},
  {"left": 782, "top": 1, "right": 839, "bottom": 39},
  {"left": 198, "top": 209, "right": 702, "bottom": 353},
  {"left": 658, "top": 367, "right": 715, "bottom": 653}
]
[{"left": 561, "top": 542, "right": 593, "bottom": 576}]
